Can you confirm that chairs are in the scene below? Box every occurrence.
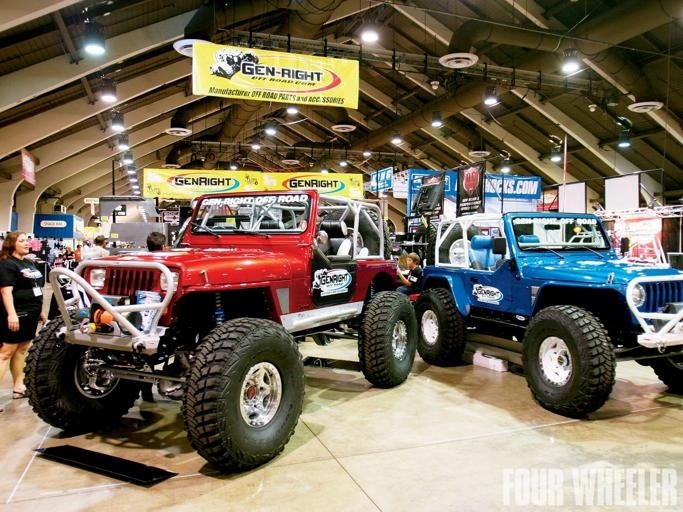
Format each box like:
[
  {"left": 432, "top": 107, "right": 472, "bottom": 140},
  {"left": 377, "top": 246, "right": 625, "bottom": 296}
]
[
  {"left": 468, "top": 232, "right": 503, "bottom": 270},
  {"left": 318, "top": 219, "right": 350, "bottom": 257},
  {"left": 518, "top": 233, "right": 539, "bottom": 244},
  {"left": 258, "top": 219, "right": 284, "bottom": 230}
]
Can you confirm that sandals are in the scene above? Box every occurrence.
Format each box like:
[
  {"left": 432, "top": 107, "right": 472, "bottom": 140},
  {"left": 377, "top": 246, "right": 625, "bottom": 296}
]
[{"left": 10, "top": 387, "right": 32, "bottom": 402}]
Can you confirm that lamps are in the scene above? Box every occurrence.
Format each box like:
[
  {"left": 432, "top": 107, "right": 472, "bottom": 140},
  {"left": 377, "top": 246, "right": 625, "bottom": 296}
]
[
  {"left": 319, "top": 45, "right": 632, "bottom": 175},
  {"left": 82, "top": 12, "right": 143, "bottom": 199}
]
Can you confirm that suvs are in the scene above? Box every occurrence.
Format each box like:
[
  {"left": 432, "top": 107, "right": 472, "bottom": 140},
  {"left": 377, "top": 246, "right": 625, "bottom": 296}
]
[
  {"left": 19, "top": 175, "right": 414, "bottom": 472},
  {"left": 409, "top": 205, "right": 683, "bottom": 417}
]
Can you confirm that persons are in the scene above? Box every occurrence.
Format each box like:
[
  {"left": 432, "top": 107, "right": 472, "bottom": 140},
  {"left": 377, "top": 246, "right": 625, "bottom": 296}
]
[
  {"left": 0, "top": 227, "right": 176, "bottom": 412},
  {"left": 396, "top": 252, "right": 423, "bottom": 295}
]
